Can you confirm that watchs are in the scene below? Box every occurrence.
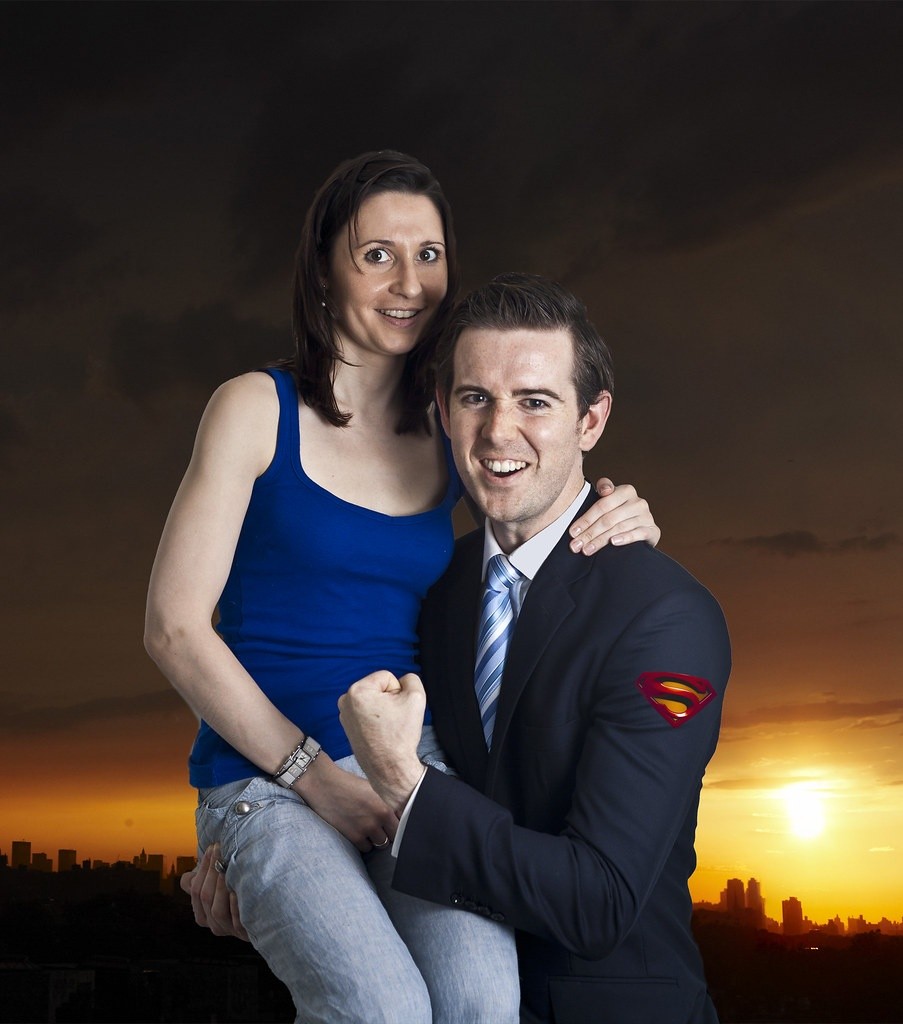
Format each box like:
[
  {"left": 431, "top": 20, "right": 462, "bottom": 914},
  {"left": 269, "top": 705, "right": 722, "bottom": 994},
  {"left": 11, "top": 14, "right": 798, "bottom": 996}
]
[{"left": 274, "top": 736, "right": 321, "bottom": 790}]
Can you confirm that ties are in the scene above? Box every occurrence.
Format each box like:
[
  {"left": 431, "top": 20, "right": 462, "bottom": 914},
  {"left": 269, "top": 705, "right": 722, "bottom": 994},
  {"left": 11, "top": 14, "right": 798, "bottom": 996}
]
[{"left": 472, "top": 554, "right": 524, "bottom": 754}]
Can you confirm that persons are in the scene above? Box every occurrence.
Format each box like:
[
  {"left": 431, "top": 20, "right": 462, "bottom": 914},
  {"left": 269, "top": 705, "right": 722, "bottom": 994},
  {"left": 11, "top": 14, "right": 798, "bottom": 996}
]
[{"left": 141, "top": 149, "right": 732, "bottom": 1024}]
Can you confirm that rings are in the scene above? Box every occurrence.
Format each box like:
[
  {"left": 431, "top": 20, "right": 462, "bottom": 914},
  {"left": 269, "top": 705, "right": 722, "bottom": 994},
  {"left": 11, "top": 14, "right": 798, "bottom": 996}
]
[{"left": 372, "top": 835, "right": 387, "bottom": 847}]
